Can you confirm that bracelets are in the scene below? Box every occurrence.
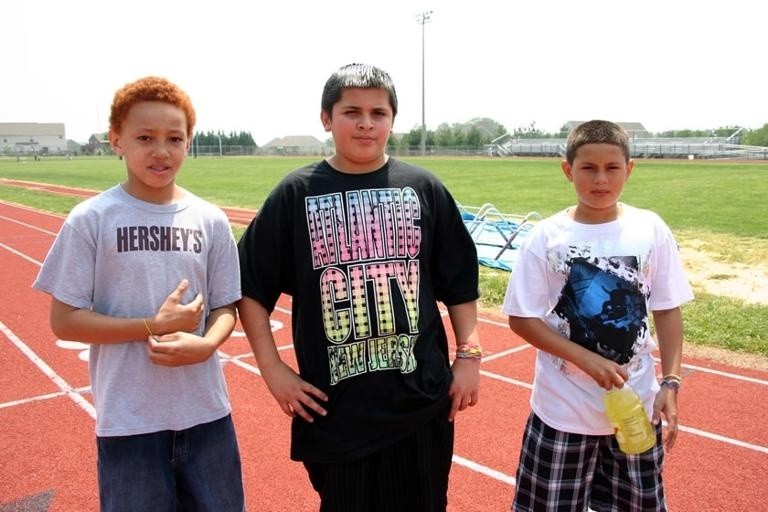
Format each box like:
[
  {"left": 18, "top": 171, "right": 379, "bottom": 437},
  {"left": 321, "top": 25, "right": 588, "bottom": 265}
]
[
  {"left": 455, "top": 343, "right": 483, "bottom": 361},
  {"left": 143, "top": 319, "right": 154, "bottom": 337},
  {"left": 660, "top": 373, "right": 682, "bottom": 390}
]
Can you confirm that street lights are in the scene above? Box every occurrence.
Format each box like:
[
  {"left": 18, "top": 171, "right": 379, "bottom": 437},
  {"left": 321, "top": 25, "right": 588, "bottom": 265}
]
[{"left": 415, "top": 9, "right": 437, "bottom": 156}]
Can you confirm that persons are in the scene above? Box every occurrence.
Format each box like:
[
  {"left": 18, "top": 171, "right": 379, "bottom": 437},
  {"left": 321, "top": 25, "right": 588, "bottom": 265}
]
[
  {"left": 223, "top": 58, "right": 488, "bottom": 510},
  {"left": 29, "top": 75, "right": 250, "bottom": 512},
  {"left": 499, "top": 116, "right": 697, "bottom": 512}
]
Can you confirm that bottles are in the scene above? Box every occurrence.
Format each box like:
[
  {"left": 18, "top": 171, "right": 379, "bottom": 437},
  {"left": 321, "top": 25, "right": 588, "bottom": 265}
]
[{"left": 605, "top": 379, "right": 656, "bottom": 456}]
